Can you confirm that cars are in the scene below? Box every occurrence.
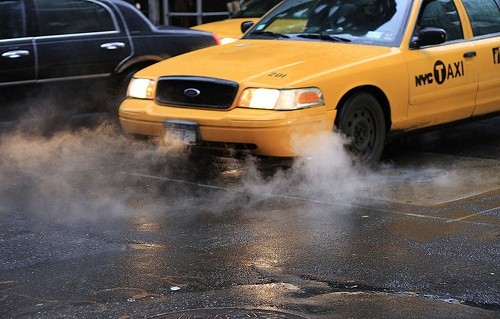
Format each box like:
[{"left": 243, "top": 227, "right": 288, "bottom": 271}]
[
  {"left": 116, "top": 0, "right": 500, "bottom": 174},
  {"left": 0, "top": 0, "right": 220, "bottom": 144}
]
